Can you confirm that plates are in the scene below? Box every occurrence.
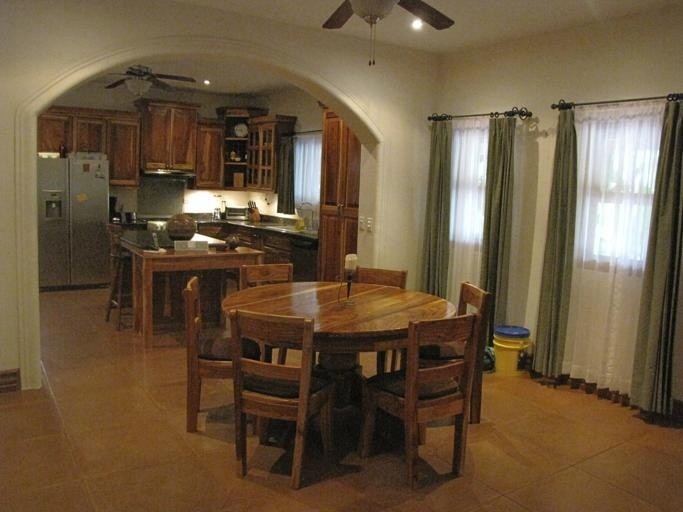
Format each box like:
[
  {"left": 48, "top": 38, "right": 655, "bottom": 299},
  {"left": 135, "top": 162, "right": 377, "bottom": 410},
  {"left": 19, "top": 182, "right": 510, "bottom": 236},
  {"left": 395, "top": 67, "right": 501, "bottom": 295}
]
[{"left": 234, "top": 123, "right": 248, "bottom": 138}]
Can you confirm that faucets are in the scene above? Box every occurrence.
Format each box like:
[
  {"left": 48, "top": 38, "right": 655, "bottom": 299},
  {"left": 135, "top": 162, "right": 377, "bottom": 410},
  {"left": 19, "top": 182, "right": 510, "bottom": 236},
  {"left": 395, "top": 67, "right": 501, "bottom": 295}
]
[{"left": 297, "top": 202, "right": 315, "bottom": 230}]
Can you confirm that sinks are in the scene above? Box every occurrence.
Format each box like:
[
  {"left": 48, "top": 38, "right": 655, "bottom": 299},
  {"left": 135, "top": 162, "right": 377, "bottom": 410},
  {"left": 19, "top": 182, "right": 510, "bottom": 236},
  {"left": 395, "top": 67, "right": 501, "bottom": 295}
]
[{"left": 295, "top": 226, "right": 318, "bottom": 231}]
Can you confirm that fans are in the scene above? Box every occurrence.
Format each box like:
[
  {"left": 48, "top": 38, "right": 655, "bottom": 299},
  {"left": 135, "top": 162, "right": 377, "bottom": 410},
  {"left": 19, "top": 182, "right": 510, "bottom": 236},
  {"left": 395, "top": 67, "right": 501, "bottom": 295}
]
[
  {"left": 103, "top": 64, "right": 197, "bottom": 91},
  {"left": 321, "top": 0, "right": 457, "bottom": 35}
]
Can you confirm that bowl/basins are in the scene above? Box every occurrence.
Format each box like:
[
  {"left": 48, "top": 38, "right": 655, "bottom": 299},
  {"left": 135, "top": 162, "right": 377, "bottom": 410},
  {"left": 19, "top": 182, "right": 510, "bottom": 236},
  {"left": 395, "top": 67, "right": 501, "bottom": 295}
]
[{"left": 127, "top": 212, "right": 136, "bottom": 220}]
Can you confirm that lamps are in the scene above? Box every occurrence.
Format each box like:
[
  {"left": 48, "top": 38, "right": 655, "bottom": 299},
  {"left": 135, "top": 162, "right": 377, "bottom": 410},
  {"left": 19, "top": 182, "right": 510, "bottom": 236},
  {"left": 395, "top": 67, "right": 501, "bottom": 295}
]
[
  {"left": 348, "top": 0, "right": 398, "bottom": 25},
  {"left": 125, "top": 79, "right": 152, "bottom": 96}
]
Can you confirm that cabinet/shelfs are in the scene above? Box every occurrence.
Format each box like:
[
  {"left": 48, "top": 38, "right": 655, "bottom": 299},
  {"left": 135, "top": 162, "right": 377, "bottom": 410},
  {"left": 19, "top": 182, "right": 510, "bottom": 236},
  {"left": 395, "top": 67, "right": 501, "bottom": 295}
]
[
  {"left": 246, "top": 113, "right": 297, "bottom": 194},
  {"left": 36, "top": 105, "right": 72, "bottom": 153},
  {"left": 184, "top": 117, "right": 223, "bottom": 191},
  {"left": 314, "top": 101, "right": 360, "bottom": 283},
  {"left": 134, "top": 98, "right": 201, "bottom": 176},
  {"left": 71, "top": 107, "right": 141, "bottom": 187},
  {"left": 259, "top": 234, "right": 291, "bottom": 284},
  {"left": 216, "top": 106, "right": 269, "bottom": 191}
]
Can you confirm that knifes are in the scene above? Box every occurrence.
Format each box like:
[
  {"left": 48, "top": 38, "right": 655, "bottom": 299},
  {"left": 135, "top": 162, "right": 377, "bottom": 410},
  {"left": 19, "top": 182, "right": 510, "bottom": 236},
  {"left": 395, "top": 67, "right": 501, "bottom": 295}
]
[{"left": 247, "top": 200, "right": 256, "bottom": 209}]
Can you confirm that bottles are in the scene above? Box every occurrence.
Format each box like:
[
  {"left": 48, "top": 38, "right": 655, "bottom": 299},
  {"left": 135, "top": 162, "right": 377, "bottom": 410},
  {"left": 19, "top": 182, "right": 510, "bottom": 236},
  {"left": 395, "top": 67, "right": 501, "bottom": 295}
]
[
  {"left": 229, "top": 150, "right": 235, "bottom": 162},
  {"left": 234, "top": 158, "right": 239, "bottom": 161},
  {"left": 214, "top": 208, "right": 220, "bottom": 220}
]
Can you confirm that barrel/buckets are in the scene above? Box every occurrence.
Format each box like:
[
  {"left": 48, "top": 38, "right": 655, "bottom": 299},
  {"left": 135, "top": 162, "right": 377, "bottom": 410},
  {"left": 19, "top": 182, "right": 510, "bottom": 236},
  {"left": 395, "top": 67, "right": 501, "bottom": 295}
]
[{"left": 492, "top": 326, "right": 532, "bottom": 377}]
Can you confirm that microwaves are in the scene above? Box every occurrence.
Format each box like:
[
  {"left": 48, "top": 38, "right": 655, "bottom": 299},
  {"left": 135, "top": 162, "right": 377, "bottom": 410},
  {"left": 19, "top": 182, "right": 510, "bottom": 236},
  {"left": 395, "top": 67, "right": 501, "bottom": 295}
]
[{"left": 225, "top": 205, "right": 248, "bottom": 222}]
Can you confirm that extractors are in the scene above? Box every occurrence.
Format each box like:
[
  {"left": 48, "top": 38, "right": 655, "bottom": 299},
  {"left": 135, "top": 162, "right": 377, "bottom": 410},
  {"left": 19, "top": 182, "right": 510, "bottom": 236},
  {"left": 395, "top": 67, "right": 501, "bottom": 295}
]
[{"left": 142, "top": 169, "right": 195, "bottom": 180}]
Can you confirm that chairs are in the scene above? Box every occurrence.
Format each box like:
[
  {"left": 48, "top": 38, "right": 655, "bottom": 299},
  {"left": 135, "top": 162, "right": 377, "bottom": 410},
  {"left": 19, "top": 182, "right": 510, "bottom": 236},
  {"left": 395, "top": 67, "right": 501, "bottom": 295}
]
[
  {"left": 351, "top": 267, "right": 407, "bottom": 376},
  {"left": 362, "top": 312, "right": 484, "bottom": 490},
  {"left": 181, "top": 273, "right": 264, "bottom": 434},
  {"left": 225, "top": 305, "right": 336, "bottom": 490},
  {"left": 401, "top": 282, "right": 494, "bottom": 426},
  {"left": 237, "top": 263, "right": 295, "bottom": 366}
]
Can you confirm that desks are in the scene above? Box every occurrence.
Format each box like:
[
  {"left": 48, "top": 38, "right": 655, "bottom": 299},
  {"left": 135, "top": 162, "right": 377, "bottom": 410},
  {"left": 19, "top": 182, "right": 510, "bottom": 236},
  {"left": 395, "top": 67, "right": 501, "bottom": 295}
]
[
  {"left": 220, "top": 280, "right": 456, "bottom": 460},
  {"left": 105, "top": 225, "right": 260, "bottom": 349}
]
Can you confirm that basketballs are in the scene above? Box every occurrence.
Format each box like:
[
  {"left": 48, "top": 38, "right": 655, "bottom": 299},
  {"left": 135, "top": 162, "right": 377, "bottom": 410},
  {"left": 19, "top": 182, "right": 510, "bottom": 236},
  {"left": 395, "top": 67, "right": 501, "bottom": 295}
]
[{"left": 166, "top": 214, "right": 196, "bottom": 241}]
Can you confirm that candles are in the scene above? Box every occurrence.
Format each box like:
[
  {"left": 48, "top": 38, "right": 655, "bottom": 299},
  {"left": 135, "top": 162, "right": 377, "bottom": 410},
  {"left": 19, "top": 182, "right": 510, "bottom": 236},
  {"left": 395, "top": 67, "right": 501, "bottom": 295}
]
[{"left": 344, "top": 253, "right": 356, "bottom": 270}]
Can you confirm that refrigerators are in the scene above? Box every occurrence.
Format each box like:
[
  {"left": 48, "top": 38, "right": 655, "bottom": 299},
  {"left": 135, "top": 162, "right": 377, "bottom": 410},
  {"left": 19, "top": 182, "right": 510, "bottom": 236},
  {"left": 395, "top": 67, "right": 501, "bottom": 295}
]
[{"left": 37, "top": 150, "right": 111, "bottom": 289}]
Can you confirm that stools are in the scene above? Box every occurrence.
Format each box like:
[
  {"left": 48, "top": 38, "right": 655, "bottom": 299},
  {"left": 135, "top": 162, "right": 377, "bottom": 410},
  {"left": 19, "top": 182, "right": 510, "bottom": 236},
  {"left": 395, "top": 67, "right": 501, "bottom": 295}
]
[{"left": 104, "top": 251, "right": 132, "bottom": 329}]
